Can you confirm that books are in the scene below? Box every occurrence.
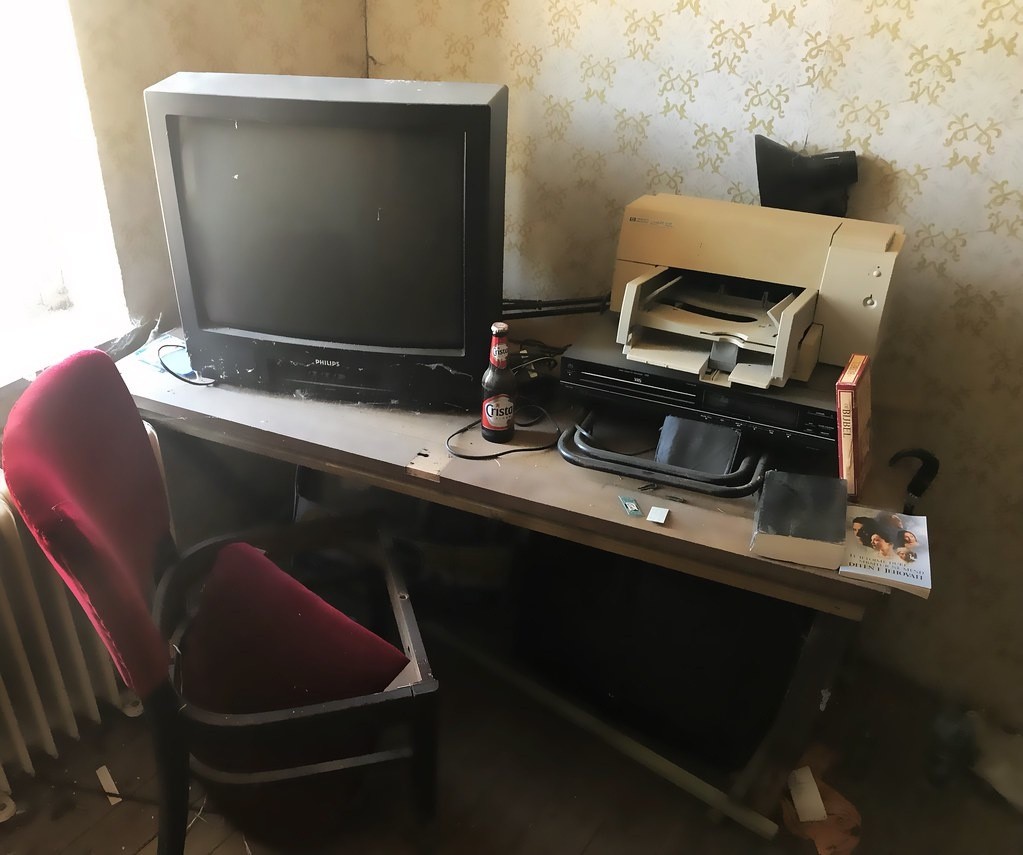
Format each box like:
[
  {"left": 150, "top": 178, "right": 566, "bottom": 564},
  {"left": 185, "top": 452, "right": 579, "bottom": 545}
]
[
  {"left": 840, "top": 505, "right": 931, "bottom": 599},
  {"left": 750, "top": 471, "right": 848, "bottom": 571}
]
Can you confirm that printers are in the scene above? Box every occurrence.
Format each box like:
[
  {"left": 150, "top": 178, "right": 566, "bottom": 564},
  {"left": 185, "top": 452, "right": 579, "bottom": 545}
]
[{"left": 608, "top": 133, "right": 911, "bottom": 388}]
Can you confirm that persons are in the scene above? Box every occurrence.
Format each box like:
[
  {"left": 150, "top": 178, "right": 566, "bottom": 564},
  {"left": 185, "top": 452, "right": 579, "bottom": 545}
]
[{"left": 853, "top": 512, "right": 917, "bottom": 563}]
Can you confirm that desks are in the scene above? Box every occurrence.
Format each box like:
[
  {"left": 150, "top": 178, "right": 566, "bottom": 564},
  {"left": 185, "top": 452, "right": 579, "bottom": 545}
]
[{"left": 108, "top": 252, "right": 894, "bottom": 838}]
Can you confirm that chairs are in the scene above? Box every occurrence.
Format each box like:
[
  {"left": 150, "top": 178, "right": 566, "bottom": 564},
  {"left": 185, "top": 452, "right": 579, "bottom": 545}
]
[{"left": 0, "top": 349, "right": 440, "bottom": 854}]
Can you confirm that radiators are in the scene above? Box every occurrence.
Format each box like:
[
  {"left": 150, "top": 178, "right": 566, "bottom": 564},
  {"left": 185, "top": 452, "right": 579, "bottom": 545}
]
[{"left": 0, "top": 417, "right": 168, "bottom": 827}]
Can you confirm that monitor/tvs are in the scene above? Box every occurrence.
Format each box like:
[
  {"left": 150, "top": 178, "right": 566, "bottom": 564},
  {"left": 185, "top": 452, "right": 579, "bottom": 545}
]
[{"left": 142, "top": 70, "right": 510, "bottom": 419}]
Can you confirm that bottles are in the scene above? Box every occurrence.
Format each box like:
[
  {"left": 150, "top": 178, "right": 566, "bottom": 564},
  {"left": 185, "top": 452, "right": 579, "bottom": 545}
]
[{"left": 481, "top": 322, "right": 514, "bottom": 444}]
